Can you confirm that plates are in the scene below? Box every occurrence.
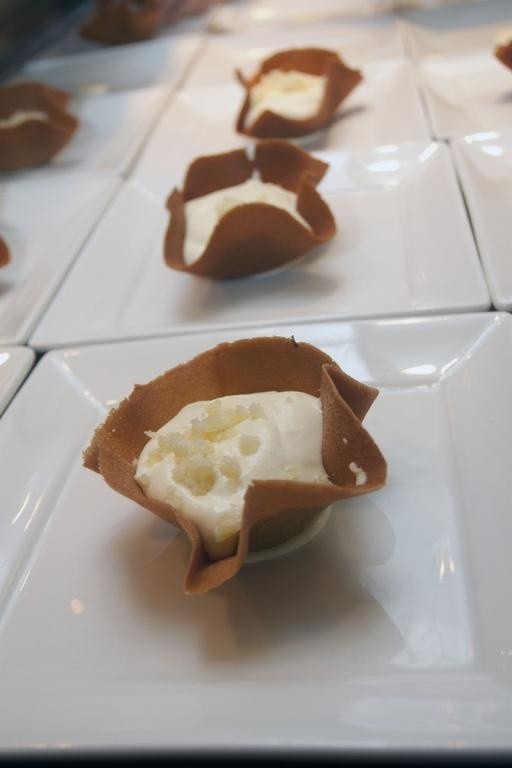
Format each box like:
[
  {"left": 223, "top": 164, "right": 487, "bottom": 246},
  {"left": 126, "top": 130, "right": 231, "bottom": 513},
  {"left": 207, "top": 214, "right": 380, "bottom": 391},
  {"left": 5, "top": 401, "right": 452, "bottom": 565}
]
[
  {"left": 26, "top": 140, "right": 494, "bottom": 348},
  {"left": 4, "top": 87, "right": 172, "bottom": 178},
  {"left": 0, "top": 345, "right": 41, "bottom": 413},
  {"left": 152, "top": 2, "right": 444, "bottom": 138},
  {"left": 8, "top": 33, "right": 203, "bottom": 88},
  {"left": 0, "top": 310, "right": 512, "bottom": 762},
  {"left": 1, "top": 176, "right": 121, "bottom": 348},
  {"left": 411, "top": 52, "right": 512, "bottom": 139},
  {"left": 446, "top": 129, "right": 511, "bottom": 313}
]
